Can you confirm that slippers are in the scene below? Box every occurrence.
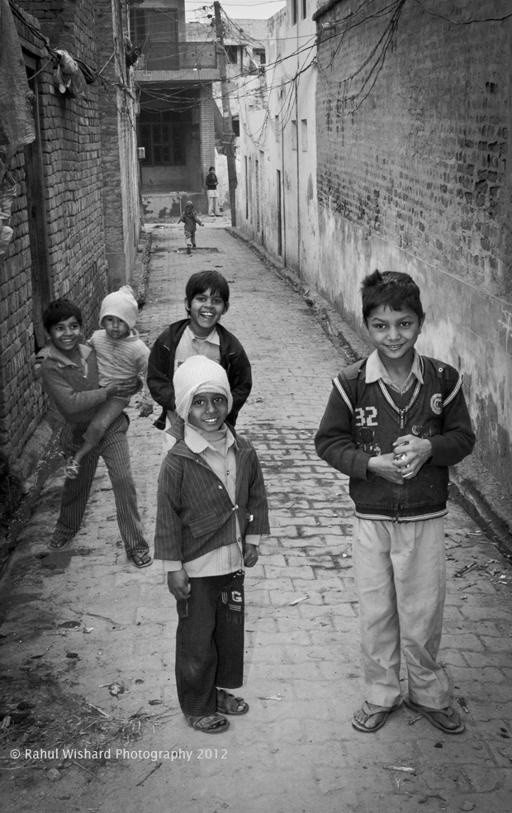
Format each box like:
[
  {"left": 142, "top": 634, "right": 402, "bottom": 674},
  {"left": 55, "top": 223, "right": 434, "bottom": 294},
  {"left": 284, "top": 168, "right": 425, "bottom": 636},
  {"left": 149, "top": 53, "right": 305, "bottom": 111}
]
[
  {"left": 47, "top": 535, "right": 69, "bottom": 550},
  {"left": 350, "top": 701, "right": 400, "bottom": 733},
  {"left": 132, "top": 551, "right": 153, "bottom": 569},
  {"left": 402, "top": 692, "right": 465, "bottom": 734}
]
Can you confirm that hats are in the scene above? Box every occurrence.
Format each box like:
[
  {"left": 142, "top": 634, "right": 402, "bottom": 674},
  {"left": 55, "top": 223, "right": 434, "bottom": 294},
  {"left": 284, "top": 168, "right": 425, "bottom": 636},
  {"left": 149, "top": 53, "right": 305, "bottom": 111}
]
[
  {"left": 172, "top": 354, "right": 233, "bottom": 421},
  {"left": 99, "top": 285, "right": 139, "bottom": 330}
]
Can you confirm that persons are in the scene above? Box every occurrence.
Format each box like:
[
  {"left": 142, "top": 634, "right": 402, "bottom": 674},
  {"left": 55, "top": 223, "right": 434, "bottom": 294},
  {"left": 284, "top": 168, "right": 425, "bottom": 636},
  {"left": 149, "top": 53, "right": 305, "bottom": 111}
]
[
  {"left": 314, "top": 266, "right": 475, "bottom": 734},
  {"left": 177, "top": 201, "right": 204, "bottom": 254},
  {"left": 154, "top": 355, "right": 272, "bottom": 734},
  {"left": 147, "top": 270, "right": 252, "bottom": 425},
  {"left": 65, "top": 285, "right": 154, "bottom": 480},
  {"left": 41, "top": 296, "right": 152, "bottom": 568},
  {"left": 205, "top": 167, "right": 223, "bottom": 216}
]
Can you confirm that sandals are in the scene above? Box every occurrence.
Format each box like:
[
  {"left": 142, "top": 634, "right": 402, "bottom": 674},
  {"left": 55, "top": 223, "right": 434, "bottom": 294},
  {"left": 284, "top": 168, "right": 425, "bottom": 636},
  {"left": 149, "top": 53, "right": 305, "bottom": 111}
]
[
  {"left": 184, "top": 710, "right": 230, "bottom": 734},
  {"left": 216, "top": 689, "right": 249, "bottom": 717},
  {"left": 64, "top": 457, "right": 80, "bottom": 479}
]
